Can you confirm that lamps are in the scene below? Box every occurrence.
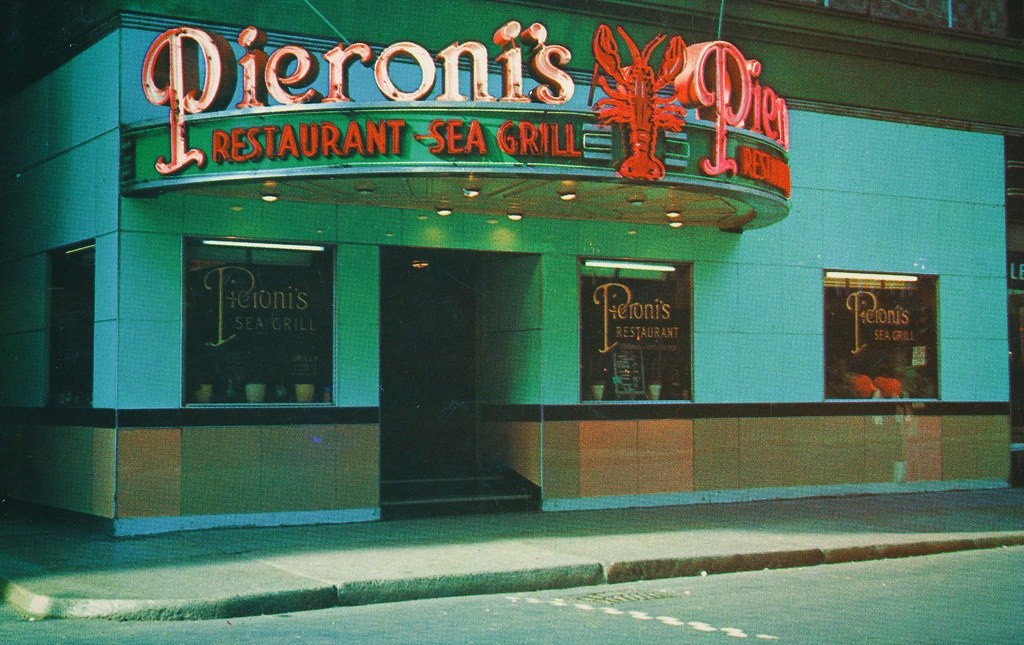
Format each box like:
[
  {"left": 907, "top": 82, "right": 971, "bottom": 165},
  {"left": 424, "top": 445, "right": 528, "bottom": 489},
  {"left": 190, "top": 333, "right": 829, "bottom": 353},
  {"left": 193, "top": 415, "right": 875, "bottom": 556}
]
[
  {"left": 66, "top": 244, "right": 95, "bottom": 255},
  {"left": 826, "top": 271, "right": 918, "bottom": 282},
  {"left": 202, "top": 239, "right": 324, "bottom": 253},
  {"left": 584, "top": 260, "right": 676, "bottom": 271}
]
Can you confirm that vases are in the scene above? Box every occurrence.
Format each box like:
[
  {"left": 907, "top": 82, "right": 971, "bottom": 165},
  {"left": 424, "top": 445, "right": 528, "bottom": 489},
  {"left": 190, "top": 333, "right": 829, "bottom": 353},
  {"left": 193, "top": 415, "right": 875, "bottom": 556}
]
[
  {"left": 591, "top": 384, "right": 604, "bottom": 400},
  {"left": 647, "top": 384, "right": 660, "bottom": 400},
  {"left": 295, "top": 383, "right": 314, "bottom": 402},
  {"left": 193, "top": 384, "right": 213, "bottom": 403},
  {"left": 244, "top": 383, "right": 266, "bottom": 403}
]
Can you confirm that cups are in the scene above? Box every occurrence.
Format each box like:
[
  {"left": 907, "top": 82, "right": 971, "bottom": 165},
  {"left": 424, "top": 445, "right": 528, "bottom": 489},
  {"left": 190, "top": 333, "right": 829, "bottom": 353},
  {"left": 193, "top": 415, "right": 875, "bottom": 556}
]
[
  {"left": 295, "top": 384, "right": 315, "bottom": 401},
  {"left": 195, "top": 382, "right": 217, "bottom": 403},
  {"left": 593, "top": 384, "right": 605, "bottom": 400},
  {"left": 246, "top": 383, "right": 268, "bottom": 403},
  {"left": 649, "top": 384, "right": 662, "bottom": 399}
]
